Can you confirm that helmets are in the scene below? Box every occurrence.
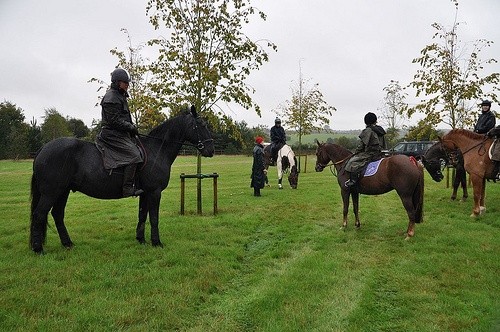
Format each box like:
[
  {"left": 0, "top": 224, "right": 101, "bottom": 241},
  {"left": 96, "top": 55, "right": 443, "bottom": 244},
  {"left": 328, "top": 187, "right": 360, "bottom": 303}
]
[
  {"left": 275, "top": 118, "right": 281, "bottom": 127},
  {"left": 110, "top": 68, "right": 131, "bottom": 83},
  {"left": 481, "top": 100, "right": 491, "bottom": 106},
  {"left": 364, "top": 112, "right": 377, "bottom": 125}
]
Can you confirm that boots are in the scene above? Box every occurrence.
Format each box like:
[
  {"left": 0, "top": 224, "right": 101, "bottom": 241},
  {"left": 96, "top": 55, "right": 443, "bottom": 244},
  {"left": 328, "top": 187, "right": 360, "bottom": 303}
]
[{"left": 122, "top": 164, "right": 144, "bottom": 197}]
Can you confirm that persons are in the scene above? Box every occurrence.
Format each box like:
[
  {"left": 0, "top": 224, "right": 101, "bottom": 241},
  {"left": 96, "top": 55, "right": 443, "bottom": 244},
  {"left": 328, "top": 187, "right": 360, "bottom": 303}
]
[
  {"left": 269, "top": 117, "right": 287, "bottom": 166},
  {"left": 343, "top": 111, "right": 388, "bottom": 187},
  {"left": 473, "top": 100, "right": 496, "bottom": 134},
  {"left": 250, "top": 136, "right": 268, "bottom": 197},
  {"left": 94, "top": 69, "right": 145, "bottom": 197}
]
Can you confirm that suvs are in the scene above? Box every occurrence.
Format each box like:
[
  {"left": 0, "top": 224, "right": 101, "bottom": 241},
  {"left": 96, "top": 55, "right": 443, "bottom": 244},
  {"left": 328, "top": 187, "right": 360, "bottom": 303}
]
[{"left": 384, "top": 141, "right": 447, "bottom": 169}]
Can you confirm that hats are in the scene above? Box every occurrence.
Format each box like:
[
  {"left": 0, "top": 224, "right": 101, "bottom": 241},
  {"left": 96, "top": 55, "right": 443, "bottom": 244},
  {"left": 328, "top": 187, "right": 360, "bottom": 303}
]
[{"left": 255, "top": 137, "right": 263, "bottom": 144}]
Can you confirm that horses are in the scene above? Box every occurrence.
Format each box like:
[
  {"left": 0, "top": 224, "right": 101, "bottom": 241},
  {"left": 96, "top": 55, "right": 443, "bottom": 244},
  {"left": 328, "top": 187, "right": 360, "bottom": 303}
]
[
  {"left": 421, "top": 141, "right": 469, "bottom": 204},
  {"left": 315, "top": 139, "right": 425, "bottom": 240},
  {"left": 252, "top": 142, "right": 299, "bottom": 189},
  {"left": 29, "top": 105, "right": 214, "bottom": 255},
  {"left": 437, "top": 126, "right": 500, "bottom": 217}
]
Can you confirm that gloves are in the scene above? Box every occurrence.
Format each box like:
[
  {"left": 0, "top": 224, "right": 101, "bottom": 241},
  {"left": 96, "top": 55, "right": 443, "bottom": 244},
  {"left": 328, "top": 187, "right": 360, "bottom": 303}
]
[{"left": 129, "top": 125, "right": 138, "bottom": 137}]
